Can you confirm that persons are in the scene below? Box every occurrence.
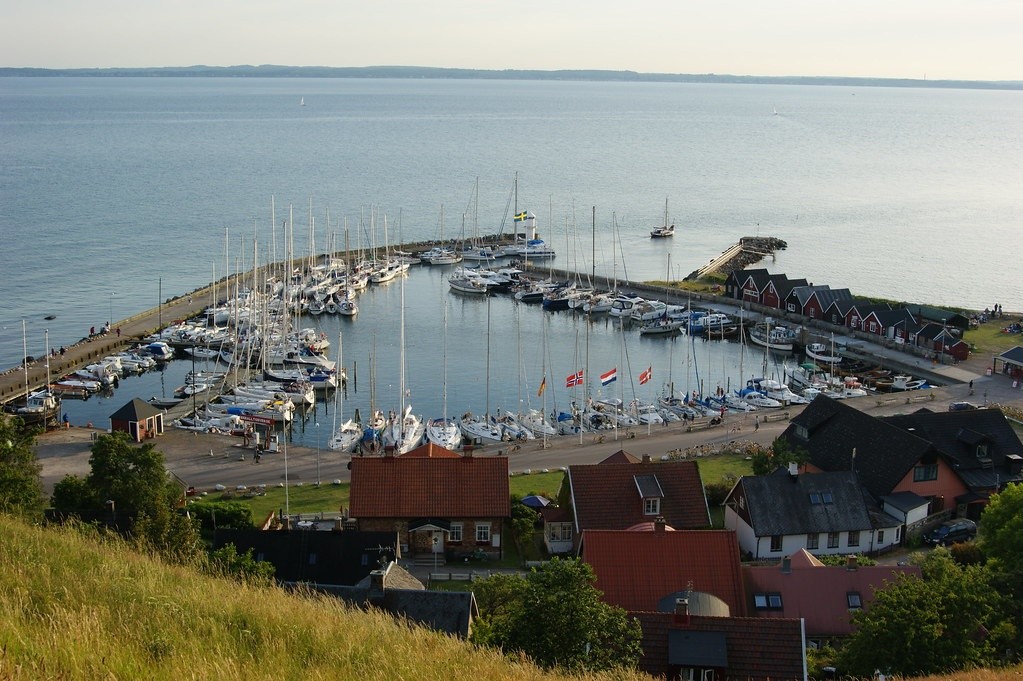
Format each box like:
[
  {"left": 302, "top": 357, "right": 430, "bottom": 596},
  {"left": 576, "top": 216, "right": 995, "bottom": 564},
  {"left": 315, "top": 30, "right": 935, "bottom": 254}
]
[
  {"left": 754, "top": 416, "right": 760, "bottom": 432},
  {"left": 681, "top": 411, "right": 687, "bottom": 426},
  {"left": 691, "top": 414, "right": 695, "bottom": 423},
  {"left": 968, "top": 380, "right": 973, "bottom": 394},
  {"left": 744, "top": 404, "right": 751, "bottom": 419},
  {"left": 116, "top": 327, "right": 121, "bottom": 337},
  {"left": 253, "top": 446, "right": 260, "bottom": 463},
  {"left": 60, "top": 346, "right": 65, "bottom": 360},
  {"left": 661, "top": 411, "right": 668, "bottom": 426},
  {"left": 720, "top": 406, "right": 724, "bottom": 418},
  {"left": 100, "top": 321, "right": 110, "bottom": 335},
  {"left": 63, "top": 413, "right": 69, "bottom": 422},
  {"left": 925, "top": 352, "right": 928, "bottom": 361},
  {"left": 90, "top": 327, "right": 94, "bottom": 335},
  {"left": 51, "top": 347, "right": 55, "bottom": 360}
]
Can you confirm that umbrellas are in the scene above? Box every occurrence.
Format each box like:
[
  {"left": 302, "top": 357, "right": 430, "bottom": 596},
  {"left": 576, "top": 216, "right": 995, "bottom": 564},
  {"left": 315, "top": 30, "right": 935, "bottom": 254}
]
[{"left": 521, "top": 496, "right": 551, "bottom": 507}]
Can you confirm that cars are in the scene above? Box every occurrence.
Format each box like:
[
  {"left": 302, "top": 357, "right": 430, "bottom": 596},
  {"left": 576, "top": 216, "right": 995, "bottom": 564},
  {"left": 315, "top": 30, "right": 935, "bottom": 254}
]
[{"left": 948, "top": 401, "right": 989, "bottom": 411}]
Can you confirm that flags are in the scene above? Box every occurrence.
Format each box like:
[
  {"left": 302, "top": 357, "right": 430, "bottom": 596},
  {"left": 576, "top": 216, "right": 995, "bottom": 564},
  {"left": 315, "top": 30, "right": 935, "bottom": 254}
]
[
  {"left": 538, "top": 378, "right": 545, "bottom": 396},
  {"left": 600, "top": 368, "right": 616, "bottom": 386},
  {"left": 566, "top": 371, "right": 584, "bottom": 388},
  {"left": 639, "top": 367, "right": 651, "bottom": 385},
  {"left": 514, "top": 211, "right": 527, "bottom": 222}
]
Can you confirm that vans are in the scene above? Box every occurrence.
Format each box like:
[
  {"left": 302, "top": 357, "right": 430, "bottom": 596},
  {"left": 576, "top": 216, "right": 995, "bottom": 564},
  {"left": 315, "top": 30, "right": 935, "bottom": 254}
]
[{"left": 926, "top": 517, "right": 978, "bottom": 549}]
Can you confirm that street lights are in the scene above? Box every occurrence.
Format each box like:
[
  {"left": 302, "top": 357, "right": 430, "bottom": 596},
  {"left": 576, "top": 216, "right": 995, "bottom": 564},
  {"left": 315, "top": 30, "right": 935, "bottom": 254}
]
[
  {"left": 110, "top": 292, "right": 115, "bottom": 331},
  {"left": 433, "top": 536, "right": 441, "bottom": 574},
  {"left": 941, "top": 318, "right": 947, "bottom": 364},
  {"left": 314, "top": 423, "right": 320, "bottom": 489}
]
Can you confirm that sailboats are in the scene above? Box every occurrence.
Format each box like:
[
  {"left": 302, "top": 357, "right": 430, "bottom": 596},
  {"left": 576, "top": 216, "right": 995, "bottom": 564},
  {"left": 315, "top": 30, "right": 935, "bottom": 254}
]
[
  {"left": 13, "top": 169, "right": 939, "bottom": 460},
  {"left": 650, "top": 195, "right": 676, "bottom": 237}
]
